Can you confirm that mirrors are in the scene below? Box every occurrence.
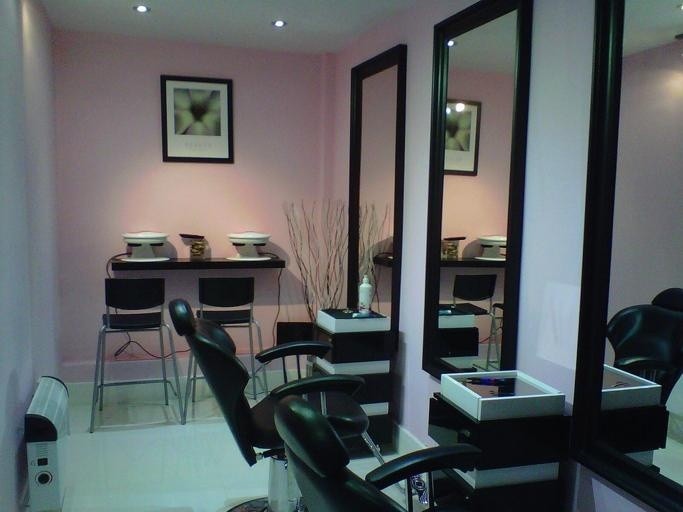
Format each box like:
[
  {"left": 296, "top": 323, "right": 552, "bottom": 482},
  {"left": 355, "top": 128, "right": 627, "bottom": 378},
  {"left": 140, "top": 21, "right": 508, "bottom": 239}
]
[
  {"left": 346, "top": 44, "right": 406, "bottom": 354},
  {"left": 421, "top": 1, "right": 533, "bottom": 384},
  {"left": 272, "top": 401, "right": 483, "bottom": 512},
  {"left": 564, "top": 1, "right": 682, "bottom": 507}
]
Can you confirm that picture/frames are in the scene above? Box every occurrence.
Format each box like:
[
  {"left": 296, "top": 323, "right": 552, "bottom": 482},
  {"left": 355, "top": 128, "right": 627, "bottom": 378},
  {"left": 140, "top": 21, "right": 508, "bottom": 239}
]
[
  {"left": 444, "top": 96, "right": 481, "bottom": 179},
  {"left": 160, "top": 75, "right": 234, "bottom": 164}
]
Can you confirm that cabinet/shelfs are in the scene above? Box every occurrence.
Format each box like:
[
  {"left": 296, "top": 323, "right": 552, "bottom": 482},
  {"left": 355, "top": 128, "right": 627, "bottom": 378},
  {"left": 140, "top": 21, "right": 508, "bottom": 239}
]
[
  {"left": 435, "top": 312, "right": 479, "bottom": 370},
  {"left": 599, "top": 360, "right": 669, "bottom": 468},
  {"left": 426, "top": 370, "right": 566, "bottom": 510},
  {"left": 315, "top": 305, "right": 391, "bottom": 447}
]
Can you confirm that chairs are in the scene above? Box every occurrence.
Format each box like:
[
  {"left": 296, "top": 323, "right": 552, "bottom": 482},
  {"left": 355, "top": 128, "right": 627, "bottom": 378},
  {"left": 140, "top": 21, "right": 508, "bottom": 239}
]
[
  {"left": 606, "top": 289, "right": 683, "bottom": 401},
  {"left": 89, "top": 276, "right": 185, "bottom": 432},
  {"left": 169, "top": 299, "right": 407, "bottom": 497},
  {"left": 438, "top": 271, "right": 501, "bottom": 369},
  {"left": 183, "top": 275, "right": 267, "bottom": 424}
]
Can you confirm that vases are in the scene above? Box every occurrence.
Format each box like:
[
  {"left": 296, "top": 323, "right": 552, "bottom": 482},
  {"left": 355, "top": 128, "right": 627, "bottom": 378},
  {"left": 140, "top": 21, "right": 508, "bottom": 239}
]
[{"left": 306, "top": 354, "right": 318, "bottom": 378}]
